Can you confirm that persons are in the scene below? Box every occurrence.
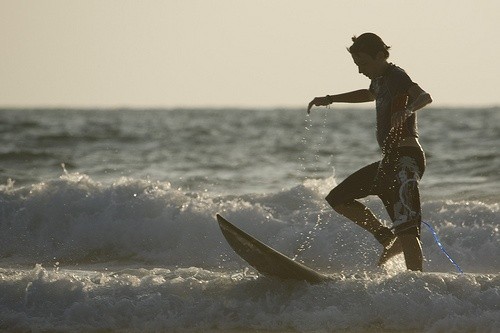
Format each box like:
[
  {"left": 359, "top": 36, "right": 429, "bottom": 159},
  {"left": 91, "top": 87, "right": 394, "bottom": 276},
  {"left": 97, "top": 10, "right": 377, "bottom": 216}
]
[{"left": 307, "top": 32, "right": 433, "bottom": 272}]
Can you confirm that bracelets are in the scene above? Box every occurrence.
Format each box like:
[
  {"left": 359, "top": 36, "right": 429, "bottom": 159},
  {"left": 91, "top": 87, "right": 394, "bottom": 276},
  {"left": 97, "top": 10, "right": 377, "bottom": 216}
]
[{"left": 327, "top": 95, "right": 332, "bottom": 104}]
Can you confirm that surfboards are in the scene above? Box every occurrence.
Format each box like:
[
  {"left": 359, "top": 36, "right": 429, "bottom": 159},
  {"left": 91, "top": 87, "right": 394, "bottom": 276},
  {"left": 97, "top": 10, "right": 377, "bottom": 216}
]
[{"left": 216, "top": 213, "right": 335, "bottom": 282}]
